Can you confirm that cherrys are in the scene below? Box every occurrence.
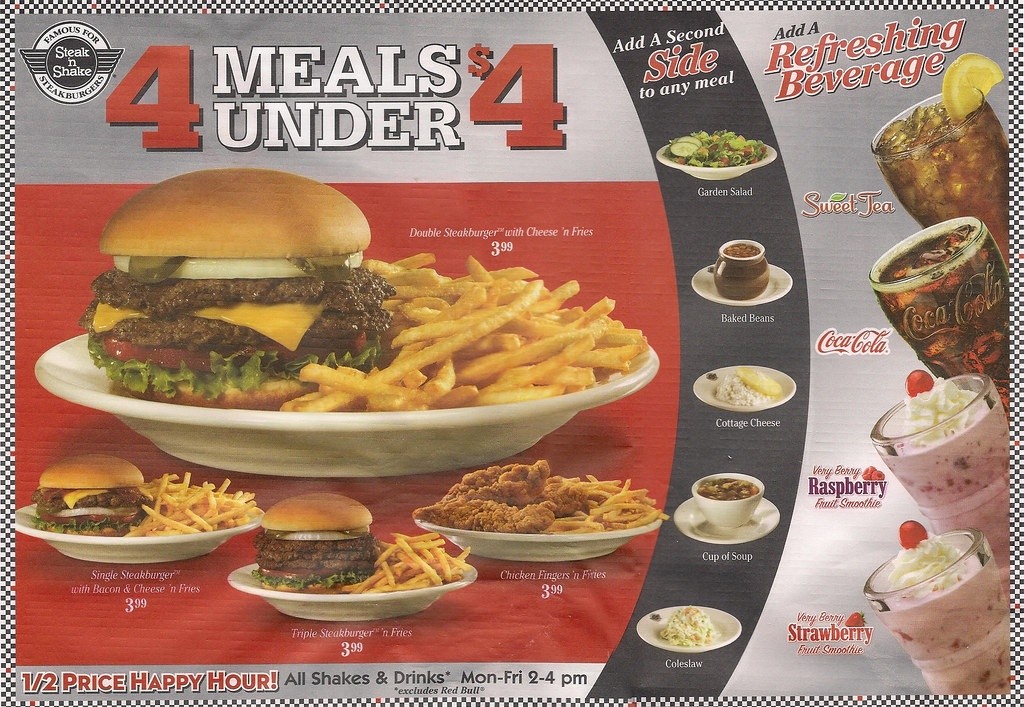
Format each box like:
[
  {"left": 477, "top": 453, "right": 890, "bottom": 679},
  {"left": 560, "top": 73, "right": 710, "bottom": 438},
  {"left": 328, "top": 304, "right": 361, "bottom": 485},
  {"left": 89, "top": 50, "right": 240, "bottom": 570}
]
[
  {"left": 898, "top": 520, "right": 928, "bottom": 549},
  {"left": 905, "top": 369, "right": 935, "bottom": 399}
]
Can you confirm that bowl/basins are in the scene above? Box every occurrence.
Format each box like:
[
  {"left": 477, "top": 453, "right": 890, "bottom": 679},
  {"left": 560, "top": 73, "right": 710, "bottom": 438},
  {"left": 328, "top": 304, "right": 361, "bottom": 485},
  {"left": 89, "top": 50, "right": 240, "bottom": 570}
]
[{"left": 692, "top": 473, "right": 764, "bottom": 528}]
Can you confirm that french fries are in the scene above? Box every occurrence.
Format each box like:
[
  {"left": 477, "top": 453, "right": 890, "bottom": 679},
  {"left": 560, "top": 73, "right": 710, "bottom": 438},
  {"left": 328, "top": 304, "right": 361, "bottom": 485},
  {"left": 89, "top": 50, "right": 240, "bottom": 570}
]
[
  {"left": 545, "top": 473, "right": 671, "bottom": 534},
  {"left": 78, "top": 168, "right": 393, "bottom": 411},
  {"left": 342, "top": 532, "right": 471, "bottom": 594},
  {"left": 281, "top": 251, "right": 651, "bottom": 413},
  {"left": 121, "top": 471, "right": 264, "bottom": 538}
]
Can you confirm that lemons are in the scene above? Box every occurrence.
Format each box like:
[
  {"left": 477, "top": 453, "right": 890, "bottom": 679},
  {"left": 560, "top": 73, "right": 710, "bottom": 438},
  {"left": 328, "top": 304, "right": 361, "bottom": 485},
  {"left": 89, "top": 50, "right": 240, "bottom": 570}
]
[{"left": 942, "top": 53, "right": 1004, "bottom": 121}]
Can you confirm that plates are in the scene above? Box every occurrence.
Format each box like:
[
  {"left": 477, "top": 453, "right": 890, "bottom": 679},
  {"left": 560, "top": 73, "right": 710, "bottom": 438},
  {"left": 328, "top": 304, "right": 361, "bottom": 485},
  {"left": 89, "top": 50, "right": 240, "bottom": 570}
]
[
  {"left": 33, "top": 332, "right": 662, "bottom": 478},
  {"left": 692, "top": 261, "right": 796, "bottom": 306},
  {"left": 655, "top": 138, "right": 778, "bottom": 181},
  {"left": 412, "top": 502, "right": 664, "bottom": 562},
  {"left": 693, "top": 365, "right": 797, "bottom": 411},
  {"left": 226, "top": 562, "right": 479, "bottom": 620},
  {"left": 674, "top": 497, "right": 782, "bottom": 544},
  {"left": 636, "top": 605, "right": 743, "bottom": 654},
  {"left": 15, "top": 503, "right": 265, "bottom": 565}
]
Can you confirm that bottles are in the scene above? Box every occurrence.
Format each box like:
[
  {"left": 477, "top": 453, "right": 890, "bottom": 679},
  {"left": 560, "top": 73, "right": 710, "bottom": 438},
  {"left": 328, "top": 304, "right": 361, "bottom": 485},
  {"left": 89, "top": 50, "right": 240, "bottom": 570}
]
[{"left": 714, "top": 239, "right": 770, "bottom": 301}]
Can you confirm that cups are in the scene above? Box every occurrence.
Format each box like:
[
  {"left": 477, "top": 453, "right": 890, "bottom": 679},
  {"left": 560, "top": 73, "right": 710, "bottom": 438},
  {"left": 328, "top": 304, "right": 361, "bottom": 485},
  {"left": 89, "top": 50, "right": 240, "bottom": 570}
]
[
  {"left": 869, "top": 215, "right": 1010, "bottom": 421},
  {"left": 870, "top": 86, "right": 1010, "bottom": 267},
  {"left": 864, "top": 526, "right": 1010, "bottom": 694},
  {"left": 869, "top": 373, "right": 1010, "bottom": 602}
]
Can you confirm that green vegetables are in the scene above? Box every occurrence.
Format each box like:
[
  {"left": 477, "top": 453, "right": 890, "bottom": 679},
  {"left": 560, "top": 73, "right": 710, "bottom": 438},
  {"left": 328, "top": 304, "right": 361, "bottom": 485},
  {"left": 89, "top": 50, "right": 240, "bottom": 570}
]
[{"left": 664, "top": 130, "right": 769, "bottom": 168}]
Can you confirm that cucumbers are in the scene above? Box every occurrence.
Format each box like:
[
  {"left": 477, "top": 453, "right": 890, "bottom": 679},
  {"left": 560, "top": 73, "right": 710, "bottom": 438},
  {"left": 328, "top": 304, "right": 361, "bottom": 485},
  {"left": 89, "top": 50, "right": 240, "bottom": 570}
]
[
  {"left": 670, "top": 142, "right": 699, "bottom": 157},
  {"left": 677, "top": 136, "right": 703, "bottom": 147}
]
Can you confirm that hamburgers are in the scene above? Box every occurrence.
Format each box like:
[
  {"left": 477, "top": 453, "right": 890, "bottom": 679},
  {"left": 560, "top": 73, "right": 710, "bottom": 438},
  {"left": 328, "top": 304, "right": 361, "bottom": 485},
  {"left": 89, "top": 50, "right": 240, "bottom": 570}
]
[
  {"left": 32, "top": 454, "right": 150, "bottom": 537},
  {"left": 252, "top": 493, "right": 380, "bottom": 594}
]
